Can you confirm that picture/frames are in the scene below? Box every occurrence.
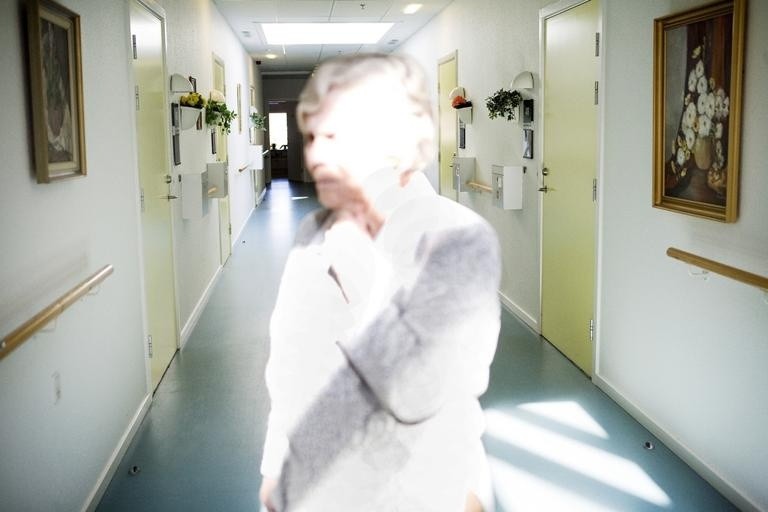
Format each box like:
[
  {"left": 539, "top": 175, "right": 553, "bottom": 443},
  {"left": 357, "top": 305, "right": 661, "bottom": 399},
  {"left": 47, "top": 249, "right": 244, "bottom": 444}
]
[
  {"left": 27, "top": 1, "right": 87, "bottom": 184},
  {"left": 190, "top": 77, "right": 202, "bottom": 129},
  {"left": 172, "top": 103, "right": 181, "bottom": 167},
  {"left": 522, "top": 100, "right": 533, "bottom": 126},
  {"left": 654, "top": 0, "right": 746, "bottom": 223},
  {"left": 522, "top": 130, "right": 532, "bottom": 160}
]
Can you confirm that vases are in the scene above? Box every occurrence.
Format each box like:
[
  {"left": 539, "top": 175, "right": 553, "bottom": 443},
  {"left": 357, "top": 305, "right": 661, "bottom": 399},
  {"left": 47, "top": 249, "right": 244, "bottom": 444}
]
[
  {"left": 454, "top": 107, "right": 472, "bottom": 125},
  {"left": 181, "top": 107, "right": 200, "bottom": 130}
]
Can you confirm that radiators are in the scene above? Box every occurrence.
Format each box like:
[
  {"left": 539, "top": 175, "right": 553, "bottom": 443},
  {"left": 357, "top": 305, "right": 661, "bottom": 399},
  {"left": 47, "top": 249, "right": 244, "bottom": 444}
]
[{"left": 251, "top": 167, "right": 267, "bottom": 208}]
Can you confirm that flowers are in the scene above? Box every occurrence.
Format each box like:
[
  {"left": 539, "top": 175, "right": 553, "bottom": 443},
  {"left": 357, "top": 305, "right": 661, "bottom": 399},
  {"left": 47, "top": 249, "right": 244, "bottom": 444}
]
[
  {"left": 206, "top": 99, "right": 237, "bottom": 137},
  {"left": 451, "top": 96, "right": 472, "bottom": 109},
  {"left": 181, "top": 93, "right": 207, "bottom": 107}
]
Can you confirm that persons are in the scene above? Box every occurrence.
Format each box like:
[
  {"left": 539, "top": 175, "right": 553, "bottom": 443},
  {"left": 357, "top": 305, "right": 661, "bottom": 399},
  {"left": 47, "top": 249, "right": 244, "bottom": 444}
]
[{"left": 256, "top": 54, "right": 501, "bottom": 509}]
